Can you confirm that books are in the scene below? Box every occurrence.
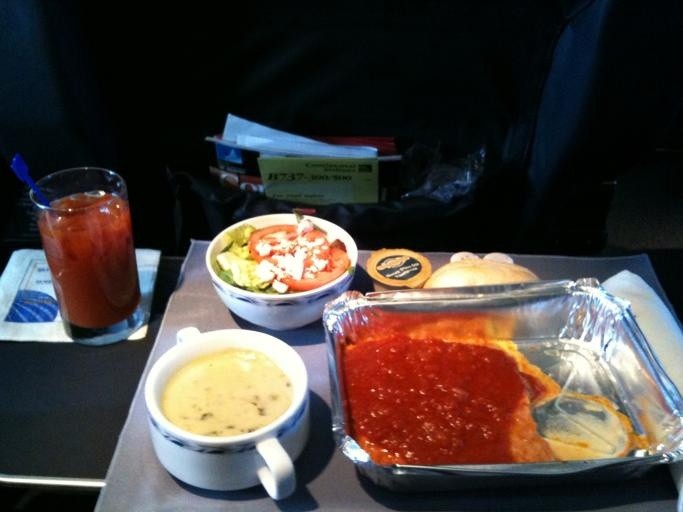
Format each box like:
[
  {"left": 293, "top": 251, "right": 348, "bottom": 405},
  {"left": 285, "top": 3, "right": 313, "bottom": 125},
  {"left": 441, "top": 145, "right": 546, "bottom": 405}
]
[{"left": 255, "top": 144, "right": 380, "bottom": 204}]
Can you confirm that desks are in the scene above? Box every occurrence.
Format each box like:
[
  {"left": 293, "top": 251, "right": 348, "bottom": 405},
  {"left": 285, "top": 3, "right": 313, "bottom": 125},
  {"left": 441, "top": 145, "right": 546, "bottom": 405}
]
[{"left": 0, "top": 247, "right": 682, "bottom": 493}]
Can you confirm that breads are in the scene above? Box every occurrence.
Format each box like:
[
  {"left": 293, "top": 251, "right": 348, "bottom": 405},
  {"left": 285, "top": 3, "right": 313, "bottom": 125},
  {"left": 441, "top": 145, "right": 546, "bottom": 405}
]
[{"left": 420, "top": 259, "right": 539, "bottom": 290}]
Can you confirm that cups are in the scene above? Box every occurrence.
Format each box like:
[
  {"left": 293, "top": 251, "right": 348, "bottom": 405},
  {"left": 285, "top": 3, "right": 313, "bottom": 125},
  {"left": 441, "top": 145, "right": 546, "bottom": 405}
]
[
  {"left": 144, "top": 326, "right": 312, "bottom": 500},
  {"left": 30, "top": 167, "right": 146, "bottom": 347}
]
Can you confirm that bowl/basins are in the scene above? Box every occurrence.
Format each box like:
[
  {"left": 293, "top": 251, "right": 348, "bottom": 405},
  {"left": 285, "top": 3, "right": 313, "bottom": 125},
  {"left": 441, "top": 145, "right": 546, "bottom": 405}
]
[{"left": 203, "top": 210, "right": 360, "bottom": 331}]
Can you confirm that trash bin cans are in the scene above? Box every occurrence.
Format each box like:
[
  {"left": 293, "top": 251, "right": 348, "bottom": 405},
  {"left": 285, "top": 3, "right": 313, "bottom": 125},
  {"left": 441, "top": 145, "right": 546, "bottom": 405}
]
[{"left": 167, "top": 93, "right": 488, "bottom": 251}]
